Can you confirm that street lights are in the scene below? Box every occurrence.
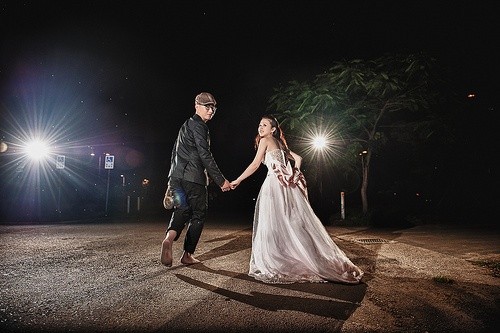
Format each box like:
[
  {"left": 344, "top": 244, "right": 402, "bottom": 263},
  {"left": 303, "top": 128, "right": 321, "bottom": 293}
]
[
  {"left": 22, "top": 132, "right": 44, "bottom": 220},
  {"left": 310, "top": 132, "right": 325, "bottom": 223}
]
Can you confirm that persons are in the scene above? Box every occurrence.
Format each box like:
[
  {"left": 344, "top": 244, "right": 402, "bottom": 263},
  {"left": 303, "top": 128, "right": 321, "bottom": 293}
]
[
  {"left": 160, "top": 91, "right": 230, "bottom": 267},
  {"left": 229, "top": 115, "right": 309, "bottom": 284}
]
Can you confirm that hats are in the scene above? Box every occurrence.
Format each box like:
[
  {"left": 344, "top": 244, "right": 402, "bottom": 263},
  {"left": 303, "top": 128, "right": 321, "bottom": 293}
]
[{"left": 195, "top": 92, "right": 217, "bottom": 106}]
